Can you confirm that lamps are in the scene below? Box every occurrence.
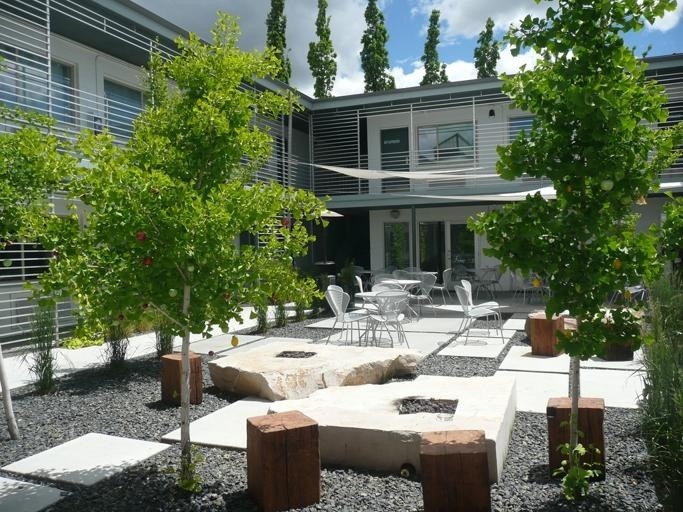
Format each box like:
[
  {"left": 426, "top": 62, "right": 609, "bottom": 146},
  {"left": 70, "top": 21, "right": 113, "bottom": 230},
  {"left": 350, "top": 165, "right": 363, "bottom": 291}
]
[{"left": 488, "top": 109, "right": 495, "bottom": 121}]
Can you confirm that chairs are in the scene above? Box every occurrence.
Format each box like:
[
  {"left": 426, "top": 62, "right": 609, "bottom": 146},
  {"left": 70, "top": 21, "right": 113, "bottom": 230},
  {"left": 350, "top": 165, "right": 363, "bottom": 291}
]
[{"left": 324, "top": 263, "right": 551, "bottom": 350}]
